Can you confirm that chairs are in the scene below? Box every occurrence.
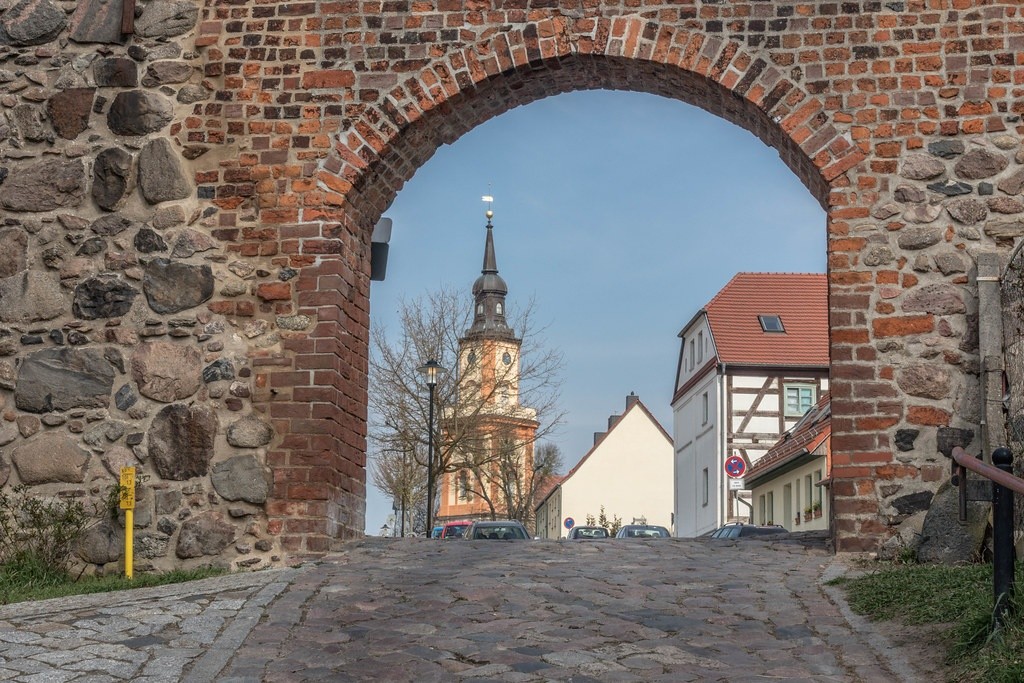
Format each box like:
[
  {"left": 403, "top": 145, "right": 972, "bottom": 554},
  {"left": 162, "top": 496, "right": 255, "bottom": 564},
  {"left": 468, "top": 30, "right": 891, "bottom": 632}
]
[
  {"left": 628, "top": 531, "right": 635, "bottom": 537},
  {"left": 594, "top": 531, "right": 601, "bottom": 536},
  {"left": 578, "top": 531, "right": 583, "bottom": 537},
  {"left": 489, "top": 533, "right": 499, "bottom": 538},
  {"left": 503, "top": 532, "right": 513, "bottom": 539},
  {"left": 652, "top": 533, "right": 660, "bottom": 536}
]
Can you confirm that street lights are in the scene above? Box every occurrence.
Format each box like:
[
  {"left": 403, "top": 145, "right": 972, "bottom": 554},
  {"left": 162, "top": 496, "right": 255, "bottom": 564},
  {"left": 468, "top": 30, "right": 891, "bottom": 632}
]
[{"left": 416, "top": 357, "right": 449, "bottom": 538}]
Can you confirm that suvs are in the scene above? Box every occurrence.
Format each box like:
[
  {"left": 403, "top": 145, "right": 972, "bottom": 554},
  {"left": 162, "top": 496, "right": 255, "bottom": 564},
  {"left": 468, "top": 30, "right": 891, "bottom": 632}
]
[{"left": 711, "top": 521, "right": 788, "bottom": 539}]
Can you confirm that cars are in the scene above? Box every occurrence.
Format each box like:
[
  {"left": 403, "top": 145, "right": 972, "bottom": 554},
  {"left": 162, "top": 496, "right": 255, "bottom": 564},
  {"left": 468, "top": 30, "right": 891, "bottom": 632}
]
[
  {"left": 615, "top": 524, "right": 671, "bottom": 540},
  {"left": 568, "top": 524, "right": 610, "bottom": 540},
  {"left": 462, "top": 519, "right": 541, "bottom": 541},
  {"left": 431, "top": 526, "right": 443, "bottom": 538},
  {"left": 441, "top": 521, "right": 473, "bottom": 539}
]
